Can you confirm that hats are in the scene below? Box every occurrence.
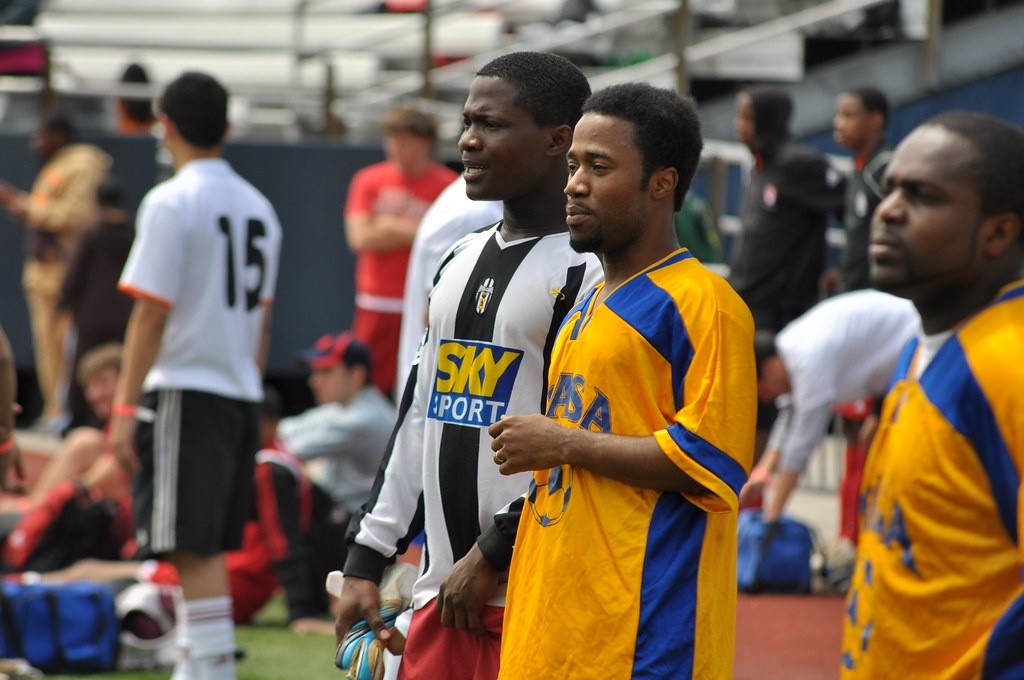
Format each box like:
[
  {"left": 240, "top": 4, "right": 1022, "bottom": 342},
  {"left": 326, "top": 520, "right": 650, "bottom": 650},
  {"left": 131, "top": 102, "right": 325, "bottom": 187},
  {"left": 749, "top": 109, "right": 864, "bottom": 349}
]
[{"left": 300, "top": 333, "right": 373, "bottom": 369}]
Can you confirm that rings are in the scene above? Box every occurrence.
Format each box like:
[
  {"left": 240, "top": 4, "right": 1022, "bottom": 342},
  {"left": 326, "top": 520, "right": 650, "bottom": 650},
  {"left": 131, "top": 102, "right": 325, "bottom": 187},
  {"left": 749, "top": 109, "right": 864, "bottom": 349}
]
[{"left": 495, "top": 450, "right": 503, "bottom": 463}]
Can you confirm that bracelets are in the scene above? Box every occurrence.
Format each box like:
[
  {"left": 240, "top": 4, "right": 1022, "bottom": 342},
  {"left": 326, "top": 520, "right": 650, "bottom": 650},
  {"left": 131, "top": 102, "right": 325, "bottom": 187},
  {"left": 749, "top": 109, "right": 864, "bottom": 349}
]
[{"left": 110, "top": 405, "right": 136, "bottom": 416}]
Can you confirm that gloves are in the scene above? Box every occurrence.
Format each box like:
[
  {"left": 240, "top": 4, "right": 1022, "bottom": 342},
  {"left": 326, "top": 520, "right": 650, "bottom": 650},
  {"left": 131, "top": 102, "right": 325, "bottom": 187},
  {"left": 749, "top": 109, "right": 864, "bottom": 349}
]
[{"left": 326, "top": 563, "right": 415, "bottom": 680}]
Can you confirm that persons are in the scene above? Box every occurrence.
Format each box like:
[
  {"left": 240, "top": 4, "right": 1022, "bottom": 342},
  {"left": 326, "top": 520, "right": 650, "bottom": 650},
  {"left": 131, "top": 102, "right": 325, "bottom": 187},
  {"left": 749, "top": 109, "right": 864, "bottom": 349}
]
[
  {"left": 487, "top": 82, "right": 757, "bottom": 680},
  {"left": 338, "top": 49, "right": 593, "bottom": 679},
  {"left": 840, "top": 111, "right": 1024, "bottom": 679},
  {"left": 108, "top": 72, "right": 281, "bottom": 680},
  {"left": 3, "top": 62, "right": 925, "bottom": 680}
]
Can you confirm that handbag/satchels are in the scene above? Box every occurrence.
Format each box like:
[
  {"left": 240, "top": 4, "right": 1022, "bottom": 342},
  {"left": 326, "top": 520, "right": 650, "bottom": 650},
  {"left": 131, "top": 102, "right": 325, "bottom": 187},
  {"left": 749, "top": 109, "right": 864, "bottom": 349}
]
[
  {"left": 736, "top": 510, "right": 812, "bottom": 594},
  {"left": 2, "top": 582, "right": 118, "bottom": 674}
]
